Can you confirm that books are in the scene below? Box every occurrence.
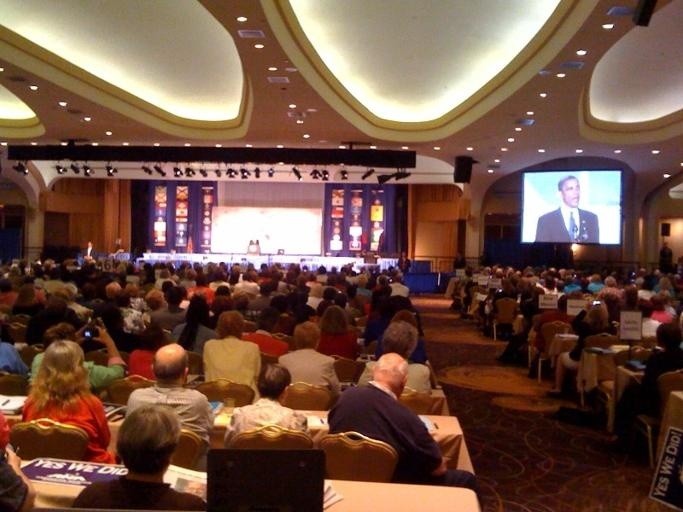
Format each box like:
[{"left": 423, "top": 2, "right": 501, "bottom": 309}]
[{"left": 322, "top": 480, "right": 343, "bottom": 509}]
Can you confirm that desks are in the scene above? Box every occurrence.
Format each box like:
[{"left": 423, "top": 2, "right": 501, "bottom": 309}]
[{"left": 21, "top": 456, "right": 484, "bottom": 512}]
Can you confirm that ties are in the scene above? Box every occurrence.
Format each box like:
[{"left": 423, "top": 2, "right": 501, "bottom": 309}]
[{"left": 568, "top": 211, "right": 576, "bottom": 242}]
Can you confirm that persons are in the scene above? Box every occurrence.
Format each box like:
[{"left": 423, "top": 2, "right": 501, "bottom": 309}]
[
  {"left": 0, "top": 410, "right": 35, "bottom": 512},
  {"left": 1, "top": 239, "right": 432, "bottom": 395},
  {"left": 70, "top": 404, "right": 207, "bottom": 511},
  {"left": 124, "top": 342, "right": 213, "bottom": 471},
  {"left": 185, "top": 479, "right": 207, "bottom": 502},
  {"left": 451, "top": 240, "right": 683, "bottom": 415},
  {"left": 534, "top": 176, "right": 599, "bottom": 243},
  {"left": 327, "top": 351, "right": 477, "bottom": 492},
  {"left": 21, "top": 339, "right": 117, "bottom": 464},
  {"left": 222, "top": 362, "right": 311, "bottom": 449}
]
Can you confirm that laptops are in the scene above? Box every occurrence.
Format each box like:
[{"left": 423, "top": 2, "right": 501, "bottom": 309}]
[{"left": 204, "top": 449, "right": 324, "bottom": 512}]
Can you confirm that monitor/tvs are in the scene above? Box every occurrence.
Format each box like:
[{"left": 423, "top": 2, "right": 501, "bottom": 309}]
[{"left": 519, "top": 167, "right": 625, "bottom": 247}]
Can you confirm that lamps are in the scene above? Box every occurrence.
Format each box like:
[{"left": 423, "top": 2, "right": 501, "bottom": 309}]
[{"left": 5, "top": 143, "right": 417, "bottom": 185}]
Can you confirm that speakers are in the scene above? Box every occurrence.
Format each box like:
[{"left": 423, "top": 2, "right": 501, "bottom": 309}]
[
  {"left": 661, "top": 223, "right": 670, "bottom": 236},
  {"left": 632, "top": 0, "right": 658, "bottom": 27},
  {"left": 454, "top": 155, "right": 473, "bottom": 184}
]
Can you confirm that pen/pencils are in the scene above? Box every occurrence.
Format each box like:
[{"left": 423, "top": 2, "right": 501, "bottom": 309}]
[
  {"left": 320, "top": 419, "right": 324, "bottom": 424},
  {"left": 2, "top": 400, "right": 10, "bottom": 406},
  {"left": 15, "top": 447, "right": 20, "bottom": 454}
]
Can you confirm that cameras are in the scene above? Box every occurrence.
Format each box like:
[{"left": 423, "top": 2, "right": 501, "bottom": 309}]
[{"left": 83, "top": 328, "right": 98, "bottom": 338}]
